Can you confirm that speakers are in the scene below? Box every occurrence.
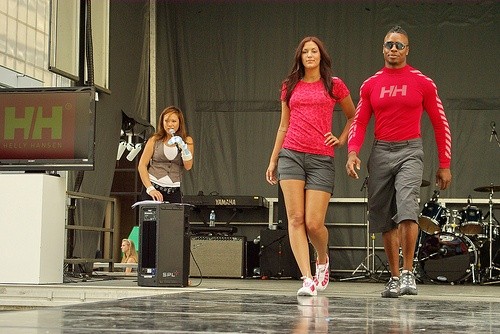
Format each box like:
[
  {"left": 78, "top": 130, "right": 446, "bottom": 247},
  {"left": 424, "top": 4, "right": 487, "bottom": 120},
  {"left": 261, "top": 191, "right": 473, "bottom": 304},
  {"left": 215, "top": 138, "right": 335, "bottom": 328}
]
[
  {"left": 191, "top": 236, "right": 247, "bottom": 278},
  {"left": 260, "top": 181, "right": 317, "bottom": 280},
  {"left": 139, "top": 204, "right": 190, "bottom": 288}
]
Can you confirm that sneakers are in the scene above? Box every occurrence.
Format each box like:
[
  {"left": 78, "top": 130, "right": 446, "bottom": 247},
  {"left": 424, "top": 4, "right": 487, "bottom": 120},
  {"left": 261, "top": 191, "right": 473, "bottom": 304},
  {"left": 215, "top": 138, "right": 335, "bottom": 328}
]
[
  {"left": 381, "top": 275, "right": 401, "bottom": 298},
  {"left": 297, "top": 276, "right": 317, "bottom": 296},
  {"left": 399, "top": 269, "right": 418, "bottom": 295},
  {"left": 313, "top": 254, "right": 329, "bottom": 291}
]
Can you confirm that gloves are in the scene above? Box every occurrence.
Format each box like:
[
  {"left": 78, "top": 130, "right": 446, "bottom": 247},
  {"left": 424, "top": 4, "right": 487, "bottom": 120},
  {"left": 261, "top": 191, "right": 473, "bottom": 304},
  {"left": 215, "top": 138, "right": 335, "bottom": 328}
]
[{"left": 168, "top": 136, "right": 192, "bottom": 161}]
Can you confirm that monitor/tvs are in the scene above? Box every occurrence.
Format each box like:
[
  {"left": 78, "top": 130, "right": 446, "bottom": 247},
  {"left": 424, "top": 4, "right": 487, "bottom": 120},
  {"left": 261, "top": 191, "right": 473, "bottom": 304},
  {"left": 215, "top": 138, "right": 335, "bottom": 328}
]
[{"left": 0, "top": 87, "right": 97, "bottom": 170}]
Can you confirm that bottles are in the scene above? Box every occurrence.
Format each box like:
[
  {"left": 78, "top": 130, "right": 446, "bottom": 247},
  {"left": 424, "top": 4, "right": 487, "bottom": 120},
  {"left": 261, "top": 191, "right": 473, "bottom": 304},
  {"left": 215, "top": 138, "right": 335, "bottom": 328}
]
[{"left": 210, "top": 210, "right": 215, "bottom": 228}]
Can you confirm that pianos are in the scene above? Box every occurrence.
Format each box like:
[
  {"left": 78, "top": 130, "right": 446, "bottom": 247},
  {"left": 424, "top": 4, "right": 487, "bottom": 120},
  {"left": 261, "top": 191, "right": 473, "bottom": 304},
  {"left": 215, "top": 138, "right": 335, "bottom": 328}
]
[{"left": 181, "top": 194, "right": 270, "bottom": 236}]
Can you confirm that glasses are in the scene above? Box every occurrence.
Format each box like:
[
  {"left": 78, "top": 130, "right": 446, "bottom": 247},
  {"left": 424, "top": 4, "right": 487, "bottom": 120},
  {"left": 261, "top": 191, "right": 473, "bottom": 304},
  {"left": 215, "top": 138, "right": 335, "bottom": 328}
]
[{"left": 383, "top": 42, "right": 408, "bottom": 50}]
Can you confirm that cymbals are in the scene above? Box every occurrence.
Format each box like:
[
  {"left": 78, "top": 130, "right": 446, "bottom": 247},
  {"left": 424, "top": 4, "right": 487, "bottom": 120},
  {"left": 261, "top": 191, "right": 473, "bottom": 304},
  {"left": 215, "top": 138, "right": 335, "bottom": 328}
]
[
  {"left": 473, "top": 185, "right": 500, "bottom": 193},
  {"left": 420, "top": 179, "right": 431, "bottom": 187}
]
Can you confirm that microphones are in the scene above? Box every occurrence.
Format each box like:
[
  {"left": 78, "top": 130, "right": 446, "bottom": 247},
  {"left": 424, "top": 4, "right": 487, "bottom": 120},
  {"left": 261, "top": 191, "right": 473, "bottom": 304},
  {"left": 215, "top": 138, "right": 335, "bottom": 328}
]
[
  {"left": 467, "top": 196, "right": 470, "bottom": 206},
  {"left": 169, "top": 130, "right": 181, "bottom": 151},
  {"left": 440, "top": 249, "right": 447, "bottom": 256},
  {"left": 490, "top": 123, "right": 495, "bottom": 142},
  {"left": 435, "top": 193, "right": 439, "bottom": 201}
]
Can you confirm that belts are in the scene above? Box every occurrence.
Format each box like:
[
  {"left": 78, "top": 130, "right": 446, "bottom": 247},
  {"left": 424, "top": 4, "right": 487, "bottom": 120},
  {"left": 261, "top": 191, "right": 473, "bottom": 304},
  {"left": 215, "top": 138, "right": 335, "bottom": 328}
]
[{"left": 151, "top": 182, "right": 180, "bottom": 194}]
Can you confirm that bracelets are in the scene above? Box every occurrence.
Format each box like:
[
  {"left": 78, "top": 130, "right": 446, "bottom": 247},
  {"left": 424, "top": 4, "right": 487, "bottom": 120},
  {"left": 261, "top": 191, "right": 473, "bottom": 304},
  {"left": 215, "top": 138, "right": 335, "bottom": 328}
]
[{"left": 145, "top": 186, "right": 156, "bottom": 195}]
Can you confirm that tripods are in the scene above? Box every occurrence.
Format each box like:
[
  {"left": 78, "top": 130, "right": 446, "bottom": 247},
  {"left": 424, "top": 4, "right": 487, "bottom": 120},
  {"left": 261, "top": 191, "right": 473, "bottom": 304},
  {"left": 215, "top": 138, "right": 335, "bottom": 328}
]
[
  {"left": 479, "top": 189, "right": 500, "bottom": 286},
  {"left": 337, "top": 211, "right": 391, "bottom": 283}
]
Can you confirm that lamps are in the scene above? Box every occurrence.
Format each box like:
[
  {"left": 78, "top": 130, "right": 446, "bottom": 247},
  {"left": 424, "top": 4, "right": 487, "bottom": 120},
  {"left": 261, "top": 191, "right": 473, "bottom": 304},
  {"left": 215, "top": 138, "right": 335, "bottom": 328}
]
[{"left": 116, "top": 133, "right": 143, "bottom": 161}]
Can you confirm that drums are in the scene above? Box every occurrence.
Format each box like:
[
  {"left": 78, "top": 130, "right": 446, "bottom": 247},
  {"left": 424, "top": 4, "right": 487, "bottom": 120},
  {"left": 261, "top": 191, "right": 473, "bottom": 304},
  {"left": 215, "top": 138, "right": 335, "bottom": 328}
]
[
  {"left": 417, "top": 232, "right": 477, "bottom": 285},
  {"left": 459, "top": 206, "right": 484, "bottom": 234},
  {"left": 418, "top": 201, "right": 447, "bottom": 235},
  {"left": 480, "top": 225, "right": 500, "bottom": 277},
  {"left": 398, "top": 230, "right": 431, "bottom": 268}
]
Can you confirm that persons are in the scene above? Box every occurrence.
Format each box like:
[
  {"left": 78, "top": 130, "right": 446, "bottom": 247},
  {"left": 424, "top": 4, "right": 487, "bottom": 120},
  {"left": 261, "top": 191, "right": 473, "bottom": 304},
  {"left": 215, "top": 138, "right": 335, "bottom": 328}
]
[
  {"left": 138, "top": 106, "right": 194, "bottom": 203},
  {"left": 346, "top": 26, "right": 451, "bottom": 298},
  {"left": 265, "top": 36, "right": 356, "bottom": 296},
  {"left": 121, "top": 239, "right": 138, "bottom": 274}
]
